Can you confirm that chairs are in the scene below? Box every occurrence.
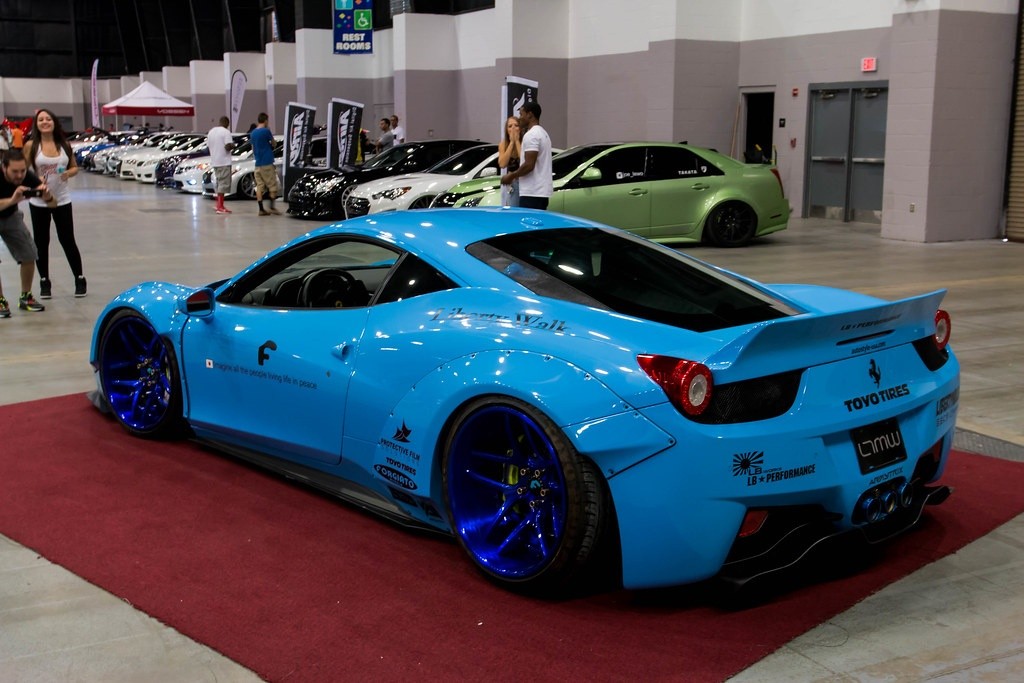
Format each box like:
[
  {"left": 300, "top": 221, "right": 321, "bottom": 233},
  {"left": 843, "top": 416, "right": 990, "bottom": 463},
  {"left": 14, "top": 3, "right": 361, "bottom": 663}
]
[{"left": 376, "top": 254, "right": 414, "bottom": 304}]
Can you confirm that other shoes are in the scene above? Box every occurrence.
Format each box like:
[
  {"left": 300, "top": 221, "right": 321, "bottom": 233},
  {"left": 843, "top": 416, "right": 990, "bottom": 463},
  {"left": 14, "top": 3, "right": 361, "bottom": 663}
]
[
  {"left": 258, "top": 210, "right": 271, "bottom": 216},
  {"left": 216, "top": 207, "right": 233, "bottom": 214},
  {"left": 270, "top": 208, "right": 282, "bottom": 215}
]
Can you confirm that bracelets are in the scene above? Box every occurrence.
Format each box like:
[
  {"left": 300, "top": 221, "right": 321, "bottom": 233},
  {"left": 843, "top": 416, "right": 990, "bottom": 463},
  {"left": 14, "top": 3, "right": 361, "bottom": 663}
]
[{"left": 47, "top": 196, "right": 53, "bottom": 202}]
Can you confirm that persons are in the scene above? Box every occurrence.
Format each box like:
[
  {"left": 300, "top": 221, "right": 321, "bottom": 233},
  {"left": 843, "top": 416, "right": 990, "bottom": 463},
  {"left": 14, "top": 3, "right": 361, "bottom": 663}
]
[
  {"left": 159, "top": 123, "right": 165, "bottom": 131},
  {"left": 11, "top": 123, "right": 23, "bottom": 148},
  {"left": 390, "top": 115, "right": 405, "bottom": 146},
  {"left": 375, "top": 119, "right": 392, "bottom": 154},
  {"left": 251, "top": 113, "right": 280, "bottom": 217},
  {"left": 357, "top": 128, "right": 371, "bottom": 163},
  {"left": 107, "top": 124, "right": 115, "bottom": 131},
  {"left": 501, "top": 102, "right": 553, "bottom": 210},
  {"left": 498, "top": 117, "right": 522, "bottom": 207},
  {"left": 247, "top": 123, "right": 256, "bottom": 140},
  {"left": 0, "top": 124, "right": 11, "bottom": 150},
  {"left": 23, "top": 109, "right": 87, "bottom": 298},
  {"left": 0, "top": 149, "right": 57, "bottom": 319},
  {"left": 206, "top": 117, "right": 237, "bottom": 214}
]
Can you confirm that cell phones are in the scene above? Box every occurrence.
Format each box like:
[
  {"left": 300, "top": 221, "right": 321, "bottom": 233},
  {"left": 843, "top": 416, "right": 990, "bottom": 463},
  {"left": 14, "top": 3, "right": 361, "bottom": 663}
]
[{"left": 22, "top": 190, "right": 42, "bottom": 197}]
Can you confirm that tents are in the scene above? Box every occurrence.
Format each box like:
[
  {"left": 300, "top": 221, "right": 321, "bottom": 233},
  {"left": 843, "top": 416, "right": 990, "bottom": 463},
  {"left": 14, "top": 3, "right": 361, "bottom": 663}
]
[{"left": 101, "top": 81, "right": 195, "bottom": 138}]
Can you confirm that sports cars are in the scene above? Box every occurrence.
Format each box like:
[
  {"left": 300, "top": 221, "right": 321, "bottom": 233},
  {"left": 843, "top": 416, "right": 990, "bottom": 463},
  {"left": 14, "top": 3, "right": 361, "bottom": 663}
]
[{"left": 90, "top": 207, "right": 960, "bottom": 597}]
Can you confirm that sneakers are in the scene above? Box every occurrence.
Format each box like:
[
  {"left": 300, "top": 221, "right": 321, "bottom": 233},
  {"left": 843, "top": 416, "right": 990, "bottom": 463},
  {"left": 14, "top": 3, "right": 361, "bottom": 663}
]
[
  {"left": 74, "top": 276, "right": 87, "bottom": 296},
  {"left": 0, "top": 296, "right": 10, "bottom": 315},
  {"left": 18, "top": 291, "right": 45, "bottom": 312},
  {"left": 39, "top": 279, "right": 52, "bottom": 298}
]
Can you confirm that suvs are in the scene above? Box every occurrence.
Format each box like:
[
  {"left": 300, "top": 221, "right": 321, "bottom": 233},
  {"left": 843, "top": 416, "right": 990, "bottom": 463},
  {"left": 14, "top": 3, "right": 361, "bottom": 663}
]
[{"left": 286, "top": 139, "right": 491, "bottom": 220}]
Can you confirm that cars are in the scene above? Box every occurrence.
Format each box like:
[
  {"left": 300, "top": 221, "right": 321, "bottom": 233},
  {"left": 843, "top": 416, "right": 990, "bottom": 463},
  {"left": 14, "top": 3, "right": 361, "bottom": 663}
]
[
  {"left": 65, "top": 128, "right": 382, "bottom": 200},
  {"left": 342, "top": 144, "right": 565, "bottom": 220},
  {"left": 428, "top": 141, "right": 794, "bottom": 248}
]
